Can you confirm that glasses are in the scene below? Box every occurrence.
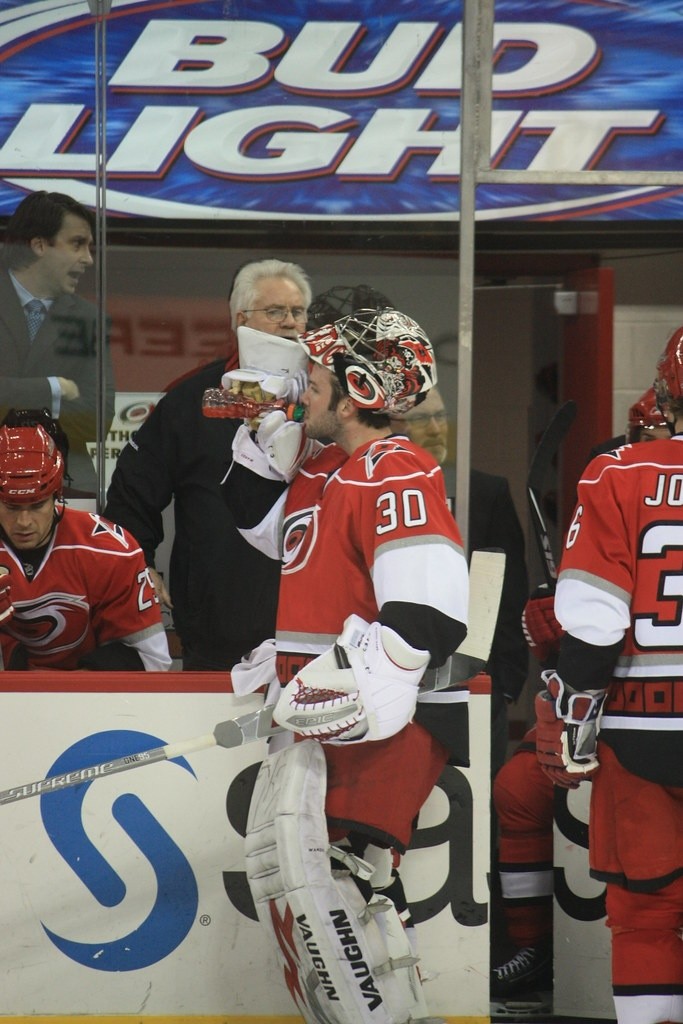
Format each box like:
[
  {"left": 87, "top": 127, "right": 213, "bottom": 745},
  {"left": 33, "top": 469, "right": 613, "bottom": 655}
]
[
  {"left": 390, "top": 409, "right": 451, "bottom": 428},
  {"left": 240, "top": 304, "right": 307, "bottom": 324}
]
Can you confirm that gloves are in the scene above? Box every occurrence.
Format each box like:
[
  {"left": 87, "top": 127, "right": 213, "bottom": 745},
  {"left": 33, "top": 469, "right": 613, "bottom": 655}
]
[
  {"left": 520, "top": 581, "right": 566, "bottom": 663},
  {"left": 533, "top": 669, "right": 611, "bottom": 790}
]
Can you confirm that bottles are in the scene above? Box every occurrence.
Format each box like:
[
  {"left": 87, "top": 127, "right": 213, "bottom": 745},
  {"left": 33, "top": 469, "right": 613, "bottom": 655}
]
[{"left": 201, "top": 387, "right": 303, "bottom": 422}]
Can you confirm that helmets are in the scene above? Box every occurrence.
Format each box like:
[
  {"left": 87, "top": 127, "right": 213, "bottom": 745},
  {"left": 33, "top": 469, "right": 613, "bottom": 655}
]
[
  {"left": 305, "top": 283, "right": 397, "bottom": 334},
  {"left": 0, "top": 425, "right": 64, "bottom": 504},
  {"left": 625, "top": 385, "right": 668, "bottom": 427},
  {"left": 652, "top": 327, "right": 682, "bottom": 415},
  {"left": 2, "top": 406, "right": 69, "bottom": 465},
  {"left": 297, "top": 306, "right": 438, "bottom": 415}
]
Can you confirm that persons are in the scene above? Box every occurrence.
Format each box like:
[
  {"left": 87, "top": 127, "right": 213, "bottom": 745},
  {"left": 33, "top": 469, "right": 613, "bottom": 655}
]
[
  {"left": 0, "top": 423, "right": 175, "bottom": 672},
  {"left": 390, "top": 386, "right": 529, "bottom": 777},
  {"left": 220, "top": 305, "right": 467, "bottom": 1023},
  {"left": 535, "top": 324, "right": 683, "bottom": 1024},
  {"left": 490, "top": 383, "right": 673, "bottom": 1000},
  {"left": 0, "top": 190, "right": 116, "bottom": 516},
  {"left": 102, "top": 258, "right": 314, "bottom": 672}
]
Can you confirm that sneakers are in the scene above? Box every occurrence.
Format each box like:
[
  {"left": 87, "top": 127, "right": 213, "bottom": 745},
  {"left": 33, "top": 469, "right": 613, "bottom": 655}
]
[{"left": 490, "top": 945, "right": 552, "bottom": 1018}]
